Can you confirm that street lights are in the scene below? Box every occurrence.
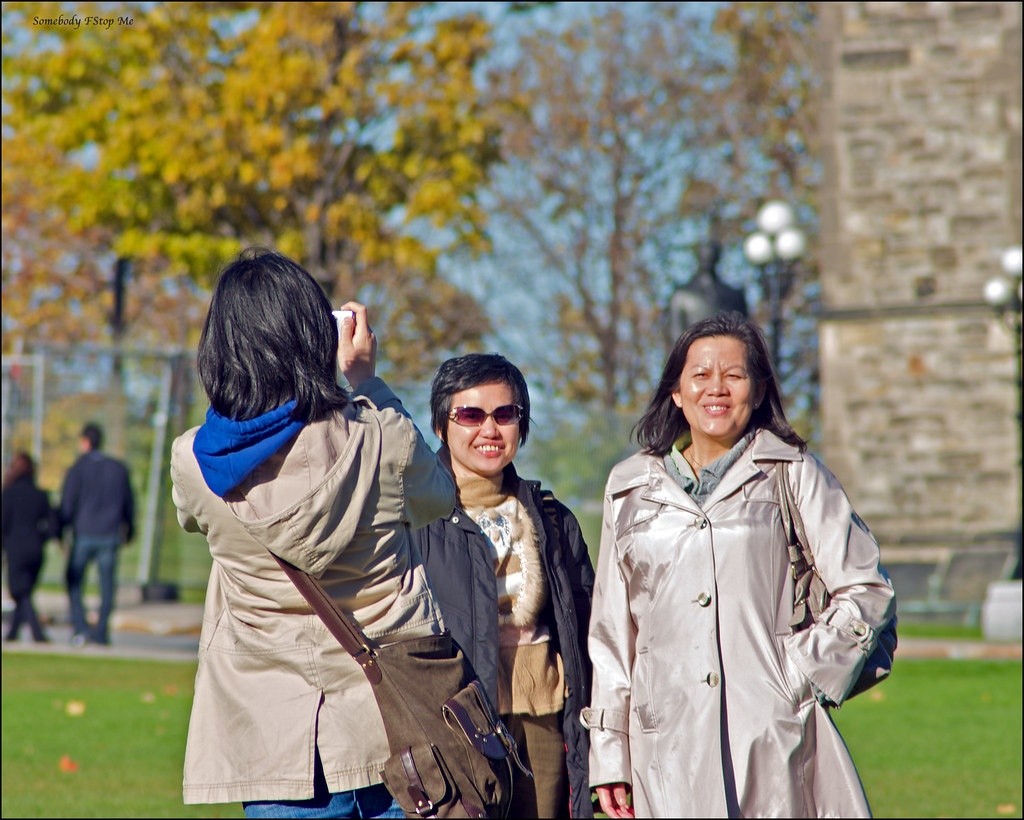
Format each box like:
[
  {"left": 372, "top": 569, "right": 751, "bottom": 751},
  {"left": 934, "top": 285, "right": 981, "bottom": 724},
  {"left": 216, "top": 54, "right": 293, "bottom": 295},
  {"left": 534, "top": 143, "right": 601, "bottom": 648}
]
[
  {"left": 745, "top": 200, "right": 804, "bottom": 372},
  {"left": 985, "top": 248, "right": 1024, "bottom": 580}
]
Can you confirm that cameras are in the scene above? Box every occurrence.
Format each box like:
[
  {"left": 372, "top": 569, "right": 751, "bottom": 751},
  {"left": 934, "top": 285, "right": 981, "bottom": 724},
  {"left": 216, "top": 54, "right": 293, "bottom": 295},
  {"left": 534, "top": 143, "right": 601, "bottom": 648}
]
[{"left": 331, "top": 311, "right": 356, "bottom": 343}]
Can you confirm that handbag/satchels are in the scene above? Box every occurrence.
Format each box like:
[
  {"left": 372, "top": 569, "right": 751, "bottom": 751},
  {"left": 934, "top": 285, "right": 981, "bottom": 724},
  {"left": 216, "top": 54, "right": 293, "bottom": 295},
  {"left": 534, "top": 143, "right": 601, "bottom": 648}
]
[
  {"left": 775, "top": 459, "right": 898, "bottom": 703},
  {"left": 362, "top": 628, "right": 533, "bottom": 820}
]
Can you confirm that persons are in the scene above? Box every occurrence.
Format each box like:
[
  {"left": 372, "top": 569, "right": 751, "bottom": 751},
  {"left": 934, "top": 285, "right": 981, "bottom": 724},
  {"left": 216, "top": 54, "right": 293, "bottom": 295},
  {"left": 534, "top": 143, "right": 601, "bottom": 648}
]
[
  {"left": 428, "top": 354, "right": 598, "bottom": 820},
  {"left": 585, "top": 319, "right": 898, "bottom": 820},
  {"left": 170, "top": 255, "right": 459, "bottom": 820},
  {"left": 57, "top": 423, "right": 135, "bottom": 646},
  {"left": 0, "top": 454, "right": 54, "bottom": 642}
]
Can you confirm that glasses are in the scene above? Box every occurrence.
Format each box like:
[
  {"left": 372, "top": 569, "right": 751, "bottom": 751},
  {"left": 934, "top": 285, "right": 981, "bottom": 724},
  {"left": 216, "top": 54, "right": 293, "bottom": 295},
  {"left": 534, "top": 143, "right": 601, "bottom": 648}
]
[{"left": 445, "top": 404, "right": 523, "bottom": 427}]
